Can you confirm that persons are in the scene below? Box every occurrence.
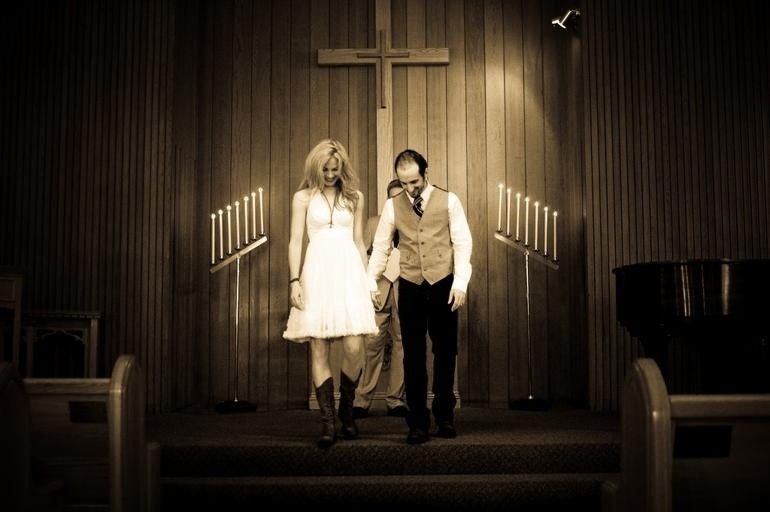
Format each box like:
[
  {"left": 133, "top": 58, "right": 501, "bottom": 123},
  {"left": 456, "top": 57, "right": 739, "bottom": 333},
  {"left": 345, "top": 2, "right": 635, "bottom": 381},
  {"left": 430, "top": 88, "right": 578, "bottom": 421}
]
[
  {"left": 280, "top": 138, "right": 381, "bottom": 447},
  {"left": 352, "top": 178, "right": 409, "bottom": 419},
  {"left": 368, "top": 147, "right": 474, "bottom": 445}
]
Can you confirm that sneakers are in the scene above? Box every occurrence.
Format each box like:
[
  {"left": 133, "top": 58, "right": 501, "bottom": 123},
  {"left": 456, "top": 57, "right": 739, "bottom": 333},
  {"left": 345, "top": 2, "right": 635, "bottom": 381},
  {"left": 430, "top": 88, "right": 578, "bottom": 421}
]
[
  {"left": 385, "top": 404, "right": 408, "bottom": 417},
  {"left": 354, "top": 405, "right": 368, "bottom": 419},
  {"left": 405, "top": 426, "right": 429, "bottom": 444},
  {"left": 435, "top": 424, "right": 457, "bottom": 438}
]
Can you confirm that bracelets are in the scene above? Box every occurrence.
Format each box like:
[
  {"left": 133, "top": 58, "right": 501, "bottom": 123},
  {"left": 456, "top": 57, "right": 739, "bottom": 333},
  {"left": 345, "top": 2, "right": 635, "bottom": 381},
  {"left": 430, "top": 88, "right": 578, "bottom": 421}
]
[{"left": 288, "top": 278, "right": 299, "bottom": 283}]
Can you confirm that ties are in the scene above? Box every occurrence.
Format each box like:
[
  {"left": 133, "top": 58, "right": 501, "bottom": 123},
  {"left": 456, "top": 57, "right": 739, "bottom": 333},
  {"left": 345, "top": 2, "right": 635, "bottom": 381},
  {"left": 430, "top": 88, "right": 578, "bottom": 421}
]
[{"left": 413, "top": 194, "right": 424, "bottom": 216}]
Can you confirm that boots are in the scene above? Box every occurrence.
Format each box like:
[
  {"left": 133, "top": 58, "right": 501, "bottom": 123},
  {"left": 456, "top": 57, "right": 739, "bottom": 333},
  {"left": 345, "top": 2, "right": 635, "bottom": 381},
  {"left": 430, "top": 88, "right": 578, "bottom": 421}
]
[
  {"left": 337, "top": 366, "right": 362, "bottom": 437},
  {"left": 312, "top": 376, "right": 338, "bottom": 446}
]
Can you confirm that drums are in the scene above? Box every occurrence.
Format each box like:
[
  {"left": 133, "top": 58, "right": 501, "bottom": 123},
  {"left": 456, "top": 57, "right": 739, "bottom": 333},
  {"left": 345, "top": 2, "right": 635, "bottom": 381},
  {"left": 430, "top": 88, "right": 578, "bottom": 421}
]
[
  {"left": 610, "top": 262, "right": 706, "bottom": 328},
  {"left": 703, "top": 256, "right": 770, "bottom": 328}
]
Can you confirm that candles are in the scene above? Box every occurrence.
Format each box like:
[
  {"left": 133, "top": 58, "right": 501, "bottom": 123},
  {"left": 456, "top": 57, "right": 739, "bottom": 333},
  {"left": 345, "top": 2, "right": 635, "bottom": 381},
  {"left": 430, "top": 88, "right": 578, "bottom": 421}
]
[
  {"left": 495, "top": 181, "right": 558, "bottom": 264},
  {"left": 209, "top": 185, "right": 265, "bottom": 264}
]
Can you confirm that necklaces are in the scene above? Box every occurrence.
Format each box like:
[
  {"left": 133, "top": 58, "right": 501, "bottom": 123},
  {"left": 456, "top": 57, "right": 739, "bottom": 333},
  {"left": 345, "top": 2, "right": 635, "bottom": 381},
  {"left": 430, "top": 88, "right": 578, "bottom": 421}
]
[{"left": 322, "top": 188, "right": 338, "bottom": 229}]
